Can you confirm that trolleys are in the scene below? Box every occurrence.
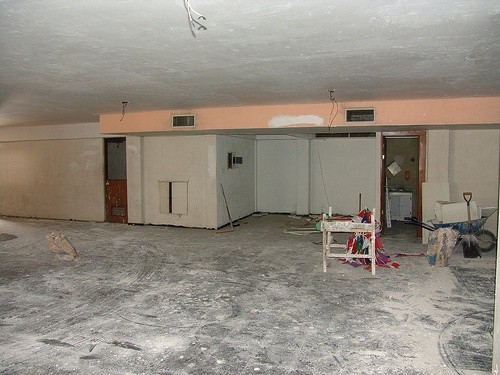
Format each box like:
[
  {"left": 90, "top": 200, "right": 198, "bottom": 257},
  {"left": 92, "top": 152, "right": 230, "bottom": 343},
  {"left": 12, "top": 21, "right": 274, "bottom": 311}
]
[{"left": 403, "top": 206, "right": 496, "bottom": 256}]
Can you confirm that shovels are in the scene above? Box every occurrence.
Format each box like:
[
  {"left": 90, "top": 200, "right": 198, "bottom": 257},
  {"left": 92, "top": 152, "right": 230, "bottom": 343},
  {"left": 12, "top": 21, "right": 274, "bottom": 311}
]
[{"left": 462, "top": 191, "right": 481, "bottom": 259}]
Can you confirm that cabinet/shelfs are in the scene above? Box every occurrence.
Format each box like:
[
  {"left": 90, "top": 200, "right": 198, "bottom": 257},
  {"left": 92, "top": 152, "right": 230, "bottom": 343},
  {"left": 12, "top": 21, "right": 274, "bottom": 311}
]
[{"left": 321, "top": 206, "right": 376, "bottom": 275}]
